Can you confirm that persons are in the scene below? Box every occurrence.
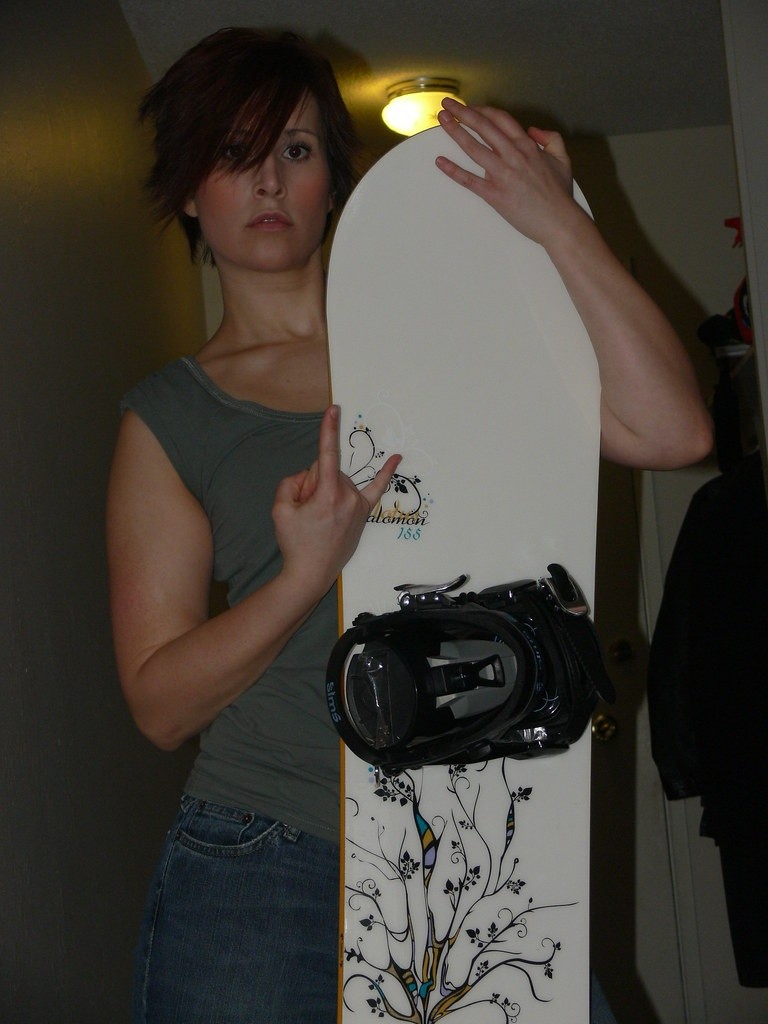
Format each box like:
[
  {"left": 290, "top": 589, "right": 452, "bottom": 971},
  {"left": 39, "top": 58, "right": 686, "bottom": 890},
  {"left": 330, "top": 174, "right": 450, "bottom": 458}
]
[{"left": 105, "top": 20, "right": 715, "bottom": 1024}]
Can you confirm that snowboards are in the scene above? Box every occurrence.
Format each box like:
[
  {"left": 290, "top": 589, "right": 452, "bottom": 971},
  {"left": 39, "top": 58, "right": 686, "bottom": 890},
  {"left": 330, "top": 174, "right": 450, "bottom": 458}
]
[{"left": 322, "top": 123, "right": 603, "bottom": 1024}]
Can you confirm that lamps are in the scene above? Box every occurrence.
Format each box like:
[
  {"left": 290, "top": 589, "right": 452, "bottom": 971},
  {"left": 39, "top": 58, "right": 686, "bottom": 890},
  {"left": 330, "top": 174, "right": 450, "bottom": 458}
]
[{"left": 382, "top": 76, "right": 467, "bottom": 137}]
